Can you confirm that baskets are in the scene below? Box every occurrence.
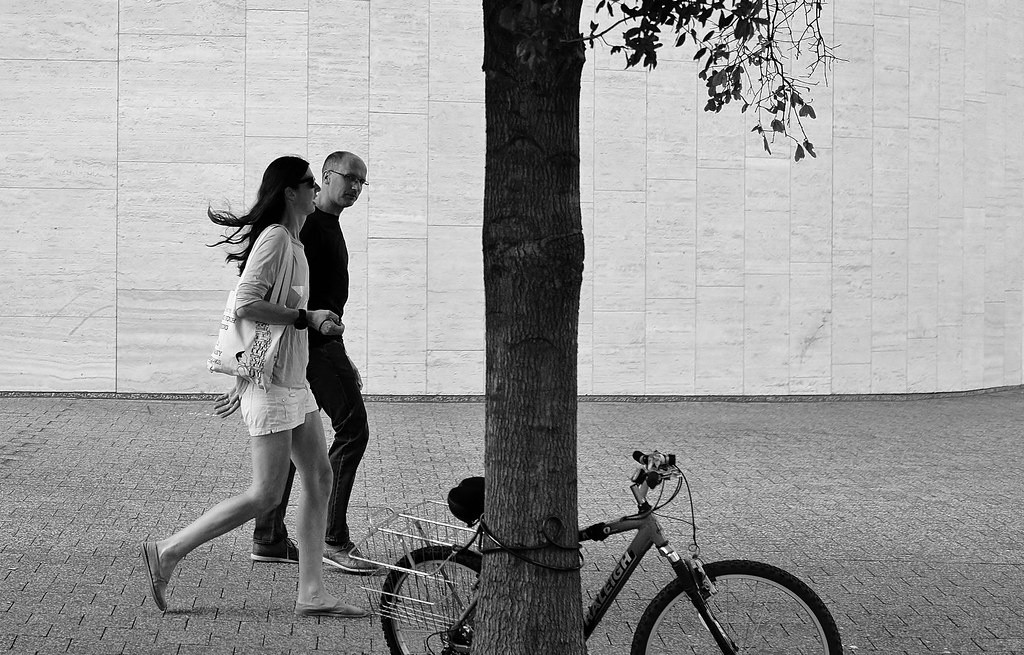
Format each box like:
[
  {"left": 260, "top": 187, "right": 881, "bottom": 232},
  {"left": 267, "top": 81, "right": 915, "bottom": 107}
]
[{"left": 348, "top": 500, "right": 484, "bottom": 632}]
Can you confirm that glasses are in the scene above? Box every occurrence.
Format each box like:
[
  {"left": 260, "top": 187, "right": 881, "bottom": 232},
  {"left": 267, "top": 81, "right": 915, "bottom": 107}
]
[
  {"left": 328, "top": 170, "right": 369, "bottom": 189},
  {"left": 299, "top": 176, "right": 315, "bottom": 188}
]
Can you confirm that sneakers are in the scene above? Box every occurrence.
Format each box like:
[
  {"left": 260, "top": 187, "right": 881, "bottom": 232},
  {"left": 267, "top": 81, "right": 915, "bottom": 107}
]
[
  {"left": 251, "top": 537, "right": 299, "bottom": 563},
  {"left": 322, "top": 537, "right": 379, "bottom": 572}
]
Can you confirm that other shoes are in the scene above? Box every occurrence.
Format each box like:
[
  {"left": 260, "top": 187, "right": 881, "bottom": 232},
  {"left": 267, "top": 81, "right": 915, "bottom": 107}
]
[
  {"left": 140, "top": 542, "right": 168, "bottom": 611},
  {"left": 296, "top": 599, "right": 368, "bottom": 618}
]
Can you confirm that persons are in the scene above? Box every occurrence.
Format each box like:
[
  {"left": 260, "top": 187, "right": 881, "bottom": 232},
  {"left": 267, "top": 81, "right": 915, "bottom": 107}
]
[
  {"left": 138, "top": 155, "right": 368, "bottom": 619},
  {"left": 251, "top": 149, "right": 382, "bottom": 577}
]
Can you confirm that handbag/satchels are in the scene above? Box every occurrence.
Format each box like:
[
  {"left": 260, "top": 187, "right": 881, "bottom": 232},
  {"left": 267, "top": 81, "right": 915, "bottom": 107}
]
[{"left": 207, "top": 224, "right": 293, "bottom": 393}]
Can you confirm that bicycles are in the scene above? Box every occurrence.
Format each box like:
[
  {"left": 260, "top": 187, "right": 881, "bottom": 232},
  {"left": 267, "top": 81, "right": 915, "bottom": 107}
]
[{"left": 346, "top": 447, "right": 845, "bottom": 655}]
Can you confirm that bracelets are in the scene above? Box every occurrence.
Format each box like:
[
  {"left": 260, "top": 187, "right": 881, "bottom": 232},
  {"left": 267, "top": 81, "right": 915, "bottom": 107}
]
[
  {"left": 293, "top": 309, "right": 309, "bottom": 331},
  {"left": 318, "top": 318, "right": 333, "bottom": 334}
]
